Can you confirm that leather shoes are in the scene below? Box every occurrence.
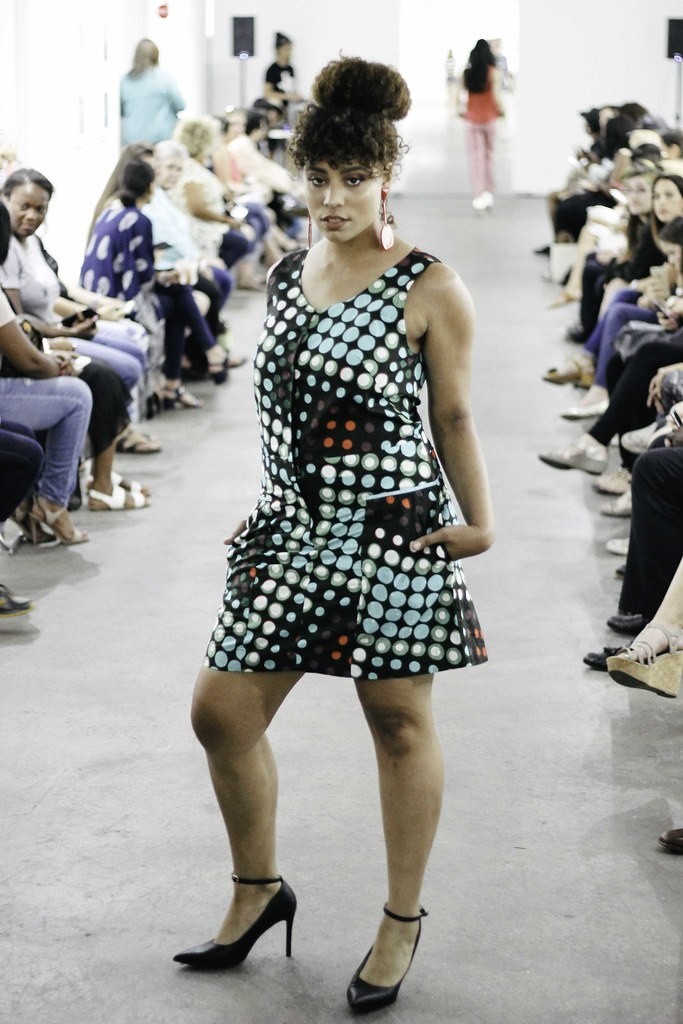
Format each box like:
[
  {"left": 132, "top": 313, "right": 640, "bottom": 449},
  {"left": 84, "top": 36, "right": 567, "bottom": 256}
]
[{"left": 607, "top": 614, "right": 649, "bottom": 635}]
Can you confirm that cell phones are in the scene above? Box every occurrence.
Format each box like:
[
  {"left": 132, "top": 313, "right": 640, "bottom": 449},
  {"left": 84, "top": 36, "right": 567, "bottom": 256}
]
[
  {"left": 61, "top": 308, "right": 100, "bottom": 328},
  {"left": 655, "top": 301, "right": 669, "bottom": 319},
  {"left": 671, "top": 410, "right": 682, "bottom": 429}
]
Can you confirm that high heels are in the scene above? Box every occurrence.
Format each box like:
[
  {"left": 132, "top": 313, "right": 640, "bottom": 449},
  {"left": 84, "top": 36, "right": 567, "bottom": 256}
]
[
  {"left": 606, "top": 623, "right": 683, "bottom": 698},
  {"left": 541, "top": 351, "right": 594, "bottom": 390},
  {"left": 173, "top": 872, "right": 297, "bottom": 969},
  {"left": 13, "top": 507, "right": 57, "bottom": 544},
  {"left": 164, "top": 386, "right": 206, "bottom": 410},
  {"left": 347, "top": 902, "right": 429, "bottom": 1012},
  {"left": 209, "top": 353, "right": 248, "bottom": 385},
  {"left": 29, "top": 492, "right": 89, "bottom": 545}
]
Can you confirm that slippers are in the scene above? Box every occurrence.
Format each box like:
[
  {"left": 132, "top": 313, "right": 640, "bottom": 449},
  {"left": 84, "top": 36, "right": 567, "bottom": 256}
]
[{"left": 545, "top": 290, "right": 582, "bottom": 308}]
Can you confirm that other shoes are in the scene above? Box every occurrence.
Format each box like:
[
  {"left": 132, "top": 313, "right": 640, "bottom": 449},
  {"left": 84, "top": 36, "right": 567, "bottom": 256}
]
[
  {"left": 605, "top": 537, "right": 629, "bottom": 556},
  {"left": 538, "top": 442, "right": 609, "bottom": 476},
  {"left": 117, "top": 430, "right": 162, "bottom": 454},
  {"left": 584, "top": 648, "right": 633, "bottom": 671},
  {"left": 593, "top": 471, "right": 632, "bottom": 496},
  {"left": 617, "top": 564, "right": 625, "bottom": 575},
  {"left": 658, "top": 828, "right": 683, "bottom": 851},
  {"left": 600, "top": 493, "right": 631, "bottom": 517},
  {"left": 567, "top": 324, "right": 587, "bottom": 344},
  {"left": 238, "top": 279, "right": 267, "bottom": 292},
  {"left": 560, "top": 398, "right": 609, "bottom": 420},
  {"left": 536, "top": 246, "right": 550, "bottom": 255},
  {"left": 611, "top": 434, "right": 619, "bottom": 446},
  {"left": 0, "top": 583, "right": 34, "bottom": 618},
  {"left": 621, "top": 422, "right": 658, "bottom": 454}
]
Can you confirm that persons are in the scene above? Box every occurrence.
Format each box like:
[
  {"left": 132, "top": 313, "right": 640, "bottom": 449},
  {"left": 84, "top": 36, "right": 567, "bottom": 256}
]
[
  {"left": 443, "top": 40, "right": 513, "bottom": 209},
  {"left": 0, "top": 33, "right": 314, "bottom": 616},
  {"left": 172, "top": 59, "right": 495, "bottom": 1014},
  {"left": 538, "top": 103, "right": 683, "bottom": 855}
]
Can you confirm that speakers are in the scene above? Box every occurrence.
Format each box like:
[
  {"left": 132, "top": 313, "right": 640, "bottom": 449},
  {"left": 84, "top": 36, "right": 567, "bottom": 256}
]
[
  {"left": 233, "top": 17, "right": 255, "bottom": 57},
  {"left": 668, "top": 19, "right": 683, "bottom": 57}
]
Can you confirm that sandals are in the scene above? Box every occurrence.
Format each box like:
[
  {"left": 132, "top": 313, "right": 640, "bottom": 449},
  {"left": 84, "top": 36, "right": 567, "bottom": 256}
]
[{"left": 85, "top": 471, "right": 151, "bottom": 511}]
